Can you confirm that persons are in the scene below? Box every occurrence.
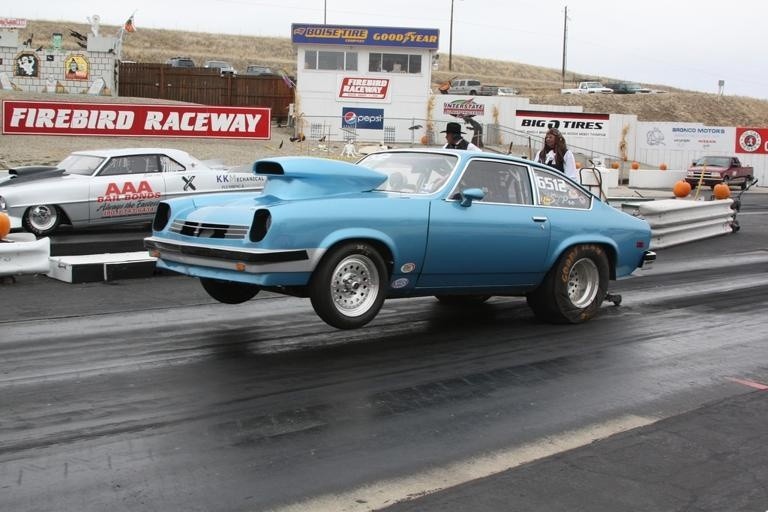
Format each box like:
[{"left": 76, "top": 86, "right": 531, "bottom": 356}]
[
  {"left": 440, "top": 123, "right": 480, "bottom": 152},
  {"left": 533, "top": 128, "right": 575, "bottom": 185}
]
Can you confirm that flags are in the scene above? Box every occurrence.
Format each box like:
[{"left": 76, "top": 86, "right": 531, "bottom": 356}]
[{"left": 124, "top": 16, "right": 137, "bottom": 33}]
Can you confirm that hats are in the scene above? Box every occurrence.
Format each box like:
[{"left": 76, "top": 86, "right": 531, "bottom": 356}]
[{"left": 440, "top": 122, "right": 467, "bottom": 135}]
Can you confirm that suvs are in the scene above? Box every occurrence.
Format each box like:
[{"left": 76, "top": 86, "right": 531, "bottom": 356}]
[
  {"left": 164, "top": 56, "right": 200, "bottom": 67},
  {"left": 240, "top": 63, "right": 276, "bottom": 76}
]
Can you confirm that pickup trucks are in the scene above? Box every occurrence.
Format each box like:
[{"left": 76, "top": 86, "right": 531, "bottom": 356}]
[
  {"left": 446, "top": 78, "right": 499, "bottom": 95},
  {"left": 561, "top": 82, "right": 614, "bottom": 95},
  {"left": 685, "top": 153, "right": 755, "bottom": 190},
  {"left": 603, "top": 81, "right": 651, "bottom": 93}
]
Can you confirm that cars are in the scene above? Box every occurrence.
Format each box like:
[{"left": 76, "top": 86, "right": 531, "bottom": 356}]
[
  {"left": 141, "top": 142, "right": 658, "bottom": 332},
  {"left": 203, "top": 62, "right": 237, "bottom": 77},
  {"left": 0, "top": 147, "right": 265, "bottom": 233}
]
